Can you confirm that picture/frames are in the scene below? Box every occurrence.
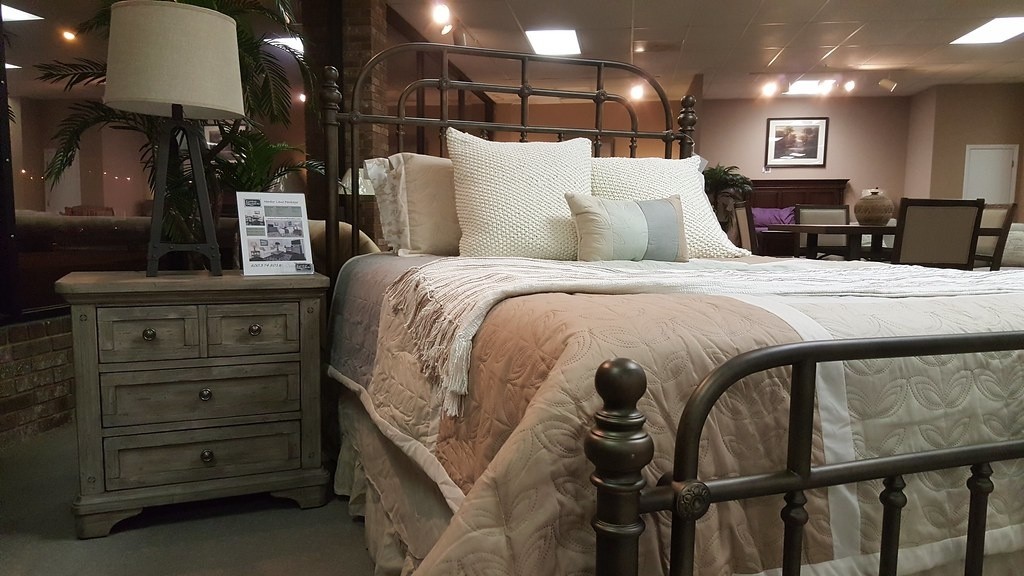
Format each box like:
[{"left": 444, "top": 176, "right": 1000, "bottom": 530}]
[
  {"left": 765, "top": 117, "right": 829, "bottom": 168},
  {"left": 203, "top": 124, "right": 248, "bottom": 164}
]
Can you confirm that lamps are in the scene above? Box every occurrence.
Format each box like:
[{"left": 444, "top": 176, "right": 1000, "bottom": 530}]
[
  {"left": 102, "top": 1, "right": 245, "bottom": 275},
  {"left": 878, "top": 71, "right": 898, "bottom": 92}
]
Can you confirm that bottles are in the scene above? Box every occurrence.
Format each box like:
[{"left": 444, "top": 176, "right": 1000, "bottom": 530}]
[{"left": 854, "top": 192, "right": 894, "bottom": 227}]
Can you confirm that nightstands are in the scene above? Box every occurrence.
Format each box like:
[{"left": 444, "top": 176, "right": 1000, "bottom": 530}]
[{"left": 54, "top": 268, "right": 330, "bottom": 539}]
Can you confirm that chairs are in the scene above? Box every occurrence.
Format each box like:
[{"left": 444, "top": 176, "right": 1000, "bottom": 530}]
[{"left": 735, "top": 197, "right": 1018, "bottom": 270}]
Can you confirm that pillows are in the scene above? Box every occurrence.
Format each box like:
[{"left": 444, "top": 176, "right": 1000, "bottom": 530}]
[{"left": 362, "top": 128, "right": 751, "bottom": 262}]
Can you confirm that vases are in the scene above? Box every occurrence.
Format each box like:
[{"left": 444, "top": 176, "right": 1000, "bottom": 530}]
[{"left": 854, "top": 192, "right": 895, "bottom": 226}]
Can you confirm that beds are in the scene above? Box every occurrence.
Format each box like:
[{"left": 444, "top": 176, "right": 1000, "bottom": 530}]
[{"left": 320, "top": 44, "right": 1023, "bottom": 576}]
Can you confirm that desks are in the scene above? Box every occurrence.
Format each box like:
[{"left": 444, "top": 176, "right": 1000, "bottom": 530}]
[
  {"left": 751, "top": 178, "right": 851, "bottom": 207},
  {"left": 768, "top": 223, "right": 896, "bottom": 262}
]
